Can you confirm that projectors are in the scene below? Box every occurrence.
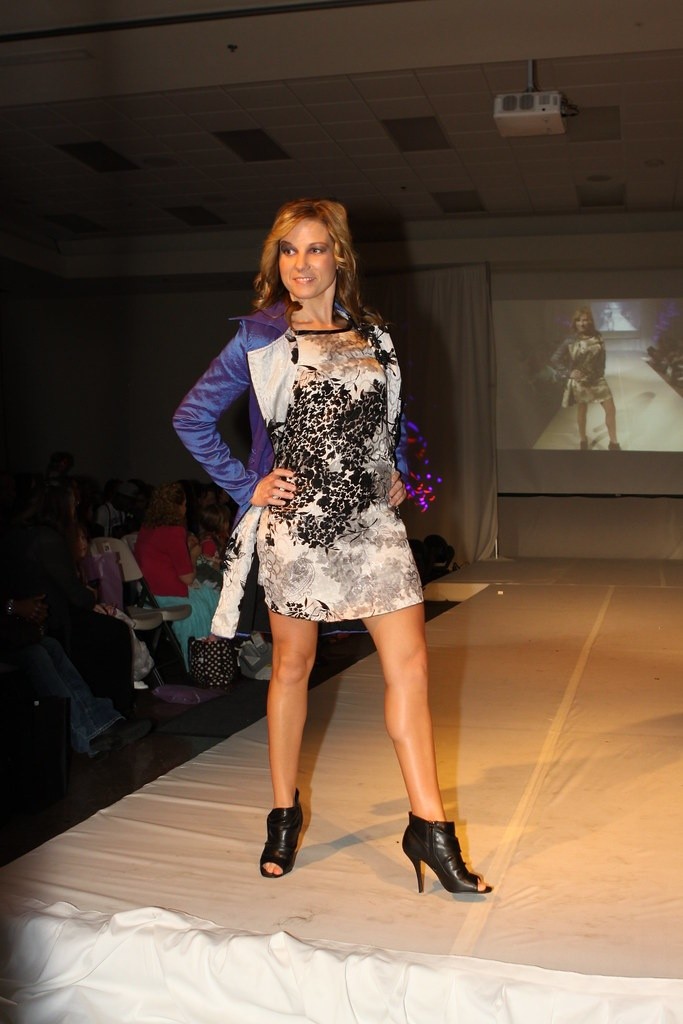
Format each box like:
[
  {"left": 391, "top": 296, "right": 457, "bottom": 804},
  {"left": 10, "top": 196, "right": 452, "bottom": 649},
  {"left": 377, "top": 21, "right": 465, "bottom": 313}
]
[{"left": 492, "top": 90, "right": 568, "bottom": 138}]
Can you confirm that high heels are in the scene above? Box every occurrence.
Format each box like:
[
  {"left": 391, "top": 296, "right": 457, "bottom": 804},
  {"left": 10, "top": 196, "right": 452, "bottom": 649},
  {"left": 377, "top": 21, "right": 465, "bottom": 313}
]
[
  {"left": 258, "top": 787, "right": 303, "bottom": 879},
  {"left": 401, "top": 811, "right": 493, "bottom": 896}
]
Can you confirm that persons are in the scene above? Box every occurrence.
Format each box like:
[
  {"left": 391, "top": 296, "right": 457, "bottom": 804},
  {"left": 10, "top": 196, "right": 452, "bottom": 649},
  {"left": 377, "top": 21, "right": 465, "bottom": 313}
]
[
  {"left": 173, "top": 202, "right": 493, "bottom": 898},
  {"left": 647, "top": 319, "right": 683, "bottom": 392},
  {"left": 0, "top": 459, "right": 273, "bottom": 800},
  {"left": 549, "top": 306, "right": 623, "bottom": 452}
]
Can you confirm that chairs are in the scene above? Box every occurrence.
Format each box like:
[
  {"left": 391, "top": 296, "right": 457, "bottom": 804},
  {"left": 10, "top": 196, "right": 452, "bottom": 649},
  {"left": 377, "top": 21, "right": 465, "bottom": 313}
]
[
  {"left": 90, "top": 537, "right": 192, "bottom": 680},
  {"left": 126, "top": 613, "right": 163, "bottom": 689},
  {"left": 123, "top": 532, "right": 157, "bottom": 608}
]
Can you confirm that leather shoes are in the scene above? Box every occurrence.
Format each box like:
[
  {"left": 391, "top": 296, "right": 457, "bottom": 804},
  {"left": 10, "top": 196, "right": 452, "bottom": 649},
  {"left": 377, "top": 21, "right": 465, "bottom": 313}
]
[{"left": 90, "top": 716, "right": 153, "bottom": 756}]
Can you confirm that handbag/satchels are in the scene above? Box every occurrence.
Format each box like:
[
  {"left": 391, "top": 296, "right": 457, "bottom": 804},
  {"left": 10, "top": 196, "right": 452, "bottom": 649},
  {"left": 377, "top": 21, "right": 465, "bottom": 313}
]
[{"left": 188, "top": 635, "right": 239, "bottom": 691}]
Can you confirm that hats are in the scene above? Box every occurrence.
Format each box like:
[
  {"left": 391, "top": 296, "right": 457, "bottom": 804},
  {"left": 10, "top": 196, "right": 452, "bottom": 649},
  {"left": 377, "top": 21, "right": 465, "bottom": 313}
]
[{"left": 116, "top": 482, "right": 144, "bottom": 502}]
